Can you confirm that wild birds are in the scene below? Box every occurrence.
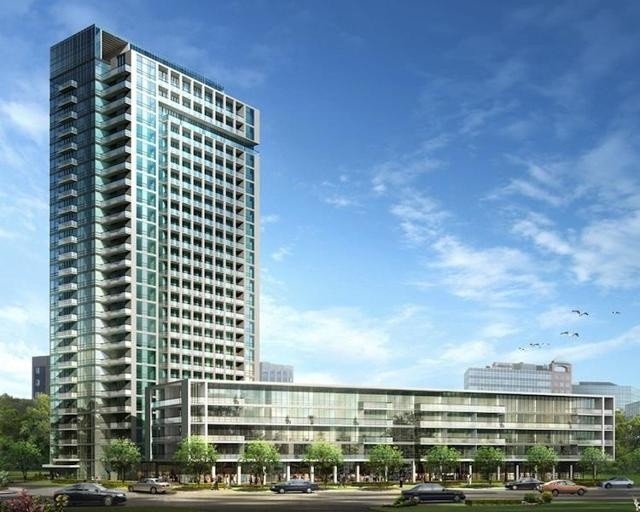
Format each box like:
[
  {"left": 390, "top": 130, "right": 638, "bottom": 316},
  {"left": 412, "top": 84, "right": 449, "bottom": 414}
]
[
  {"left": 572, "top": 310, "right": 589, "bottom": 317},
  {"left": 612, "top": 312, "right": 620, "bottom": 314},
  {"left": 560, "top": 332, "right": 579, "bottom": 337}
]
